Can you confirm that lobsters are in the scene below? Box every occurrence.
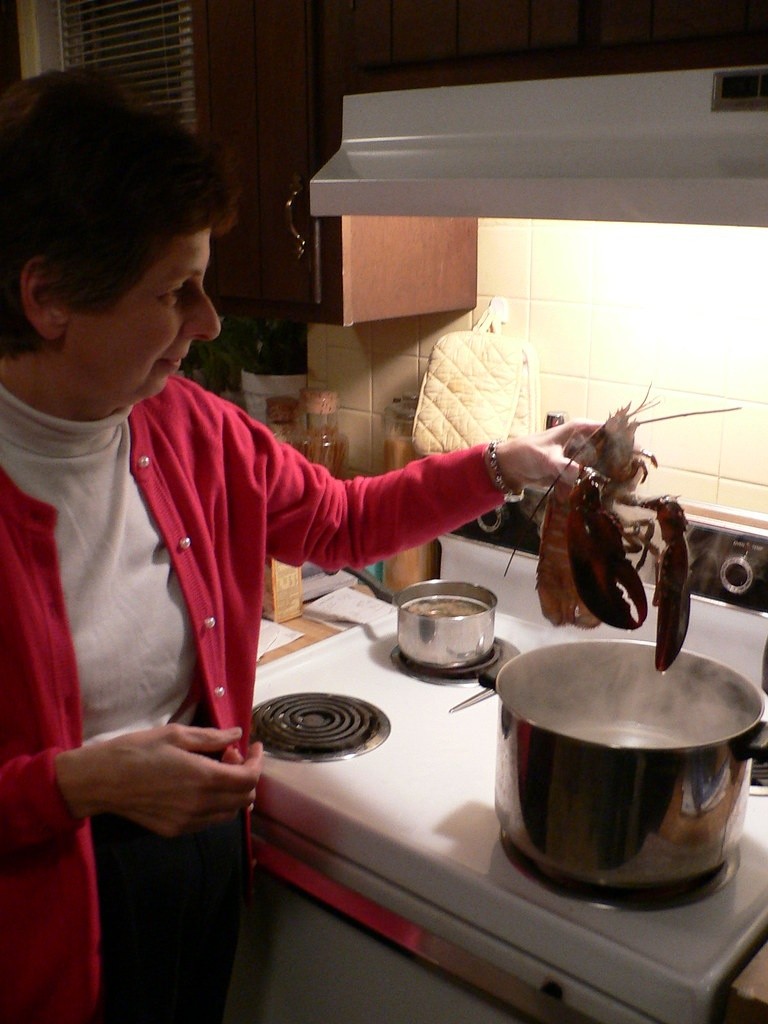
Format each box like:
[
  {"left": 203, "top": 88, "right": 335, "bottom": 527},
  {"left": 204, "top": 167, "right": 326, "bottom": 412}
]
[{"left": 502, "top": 382, "right": 742, "bottom": 673}]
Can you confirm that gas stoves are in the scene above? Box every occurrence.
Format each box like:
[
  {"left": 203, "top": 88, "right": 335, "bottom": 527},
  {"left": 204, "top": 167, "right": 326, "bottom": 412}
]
[{"left": 250, "top": 483, "right": 768, "bottom": 1024}]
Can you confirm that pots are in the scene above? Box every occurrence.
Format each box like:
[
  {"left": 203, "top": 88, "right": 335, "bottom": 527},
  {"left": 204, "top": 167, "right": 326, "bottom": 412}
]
[
  {"left": 343, "top": 564, "right": 497, "bottom": 669},
  {"left": 476, "top": 640, "right": 768, "bottom": 887}
]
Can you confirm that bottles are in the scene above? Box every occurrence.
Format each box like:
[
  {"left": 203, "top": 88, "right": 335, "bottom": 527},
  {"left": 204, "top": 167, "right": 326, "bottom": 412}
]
[
  {"left": 292, "top": 386, "right": 349, "bottom": 482},
  {"left": 263, "top": 395, "right": 303, "bottom": 454}
]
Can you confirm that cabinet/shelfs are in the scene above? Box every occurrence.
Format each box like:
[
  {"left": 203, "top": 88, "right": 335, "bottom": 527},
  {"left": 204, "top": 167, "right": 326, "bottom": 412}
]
[
  {"left": 191, "top": 0, "right": 342, "bottom": 324},
  {"left": 343, "top": 0, "right": 767, "bottom": 95}
]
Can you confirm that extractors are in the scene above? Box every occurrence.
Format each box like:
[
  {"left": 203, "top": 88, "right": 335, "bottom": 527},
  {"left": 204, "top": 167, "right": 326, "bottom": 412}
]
[{"left": 308, "top": 63, "right": 768, "bottom": 230}]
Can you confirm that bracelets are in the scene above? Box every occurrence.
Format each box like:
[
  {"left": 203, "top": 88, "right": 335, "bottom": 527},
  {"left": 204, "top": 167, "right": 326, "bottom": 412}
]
[{"left": 487, "top": 440, "right": 526, "bottom": 504}]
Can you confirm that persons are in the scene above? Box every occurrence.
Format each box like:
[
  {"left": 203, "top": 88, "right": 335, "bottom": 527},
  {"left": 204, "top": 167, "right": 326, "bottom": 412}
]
[{"left": 0, "top": 70, "right": 647, "bottom": 1024}]
